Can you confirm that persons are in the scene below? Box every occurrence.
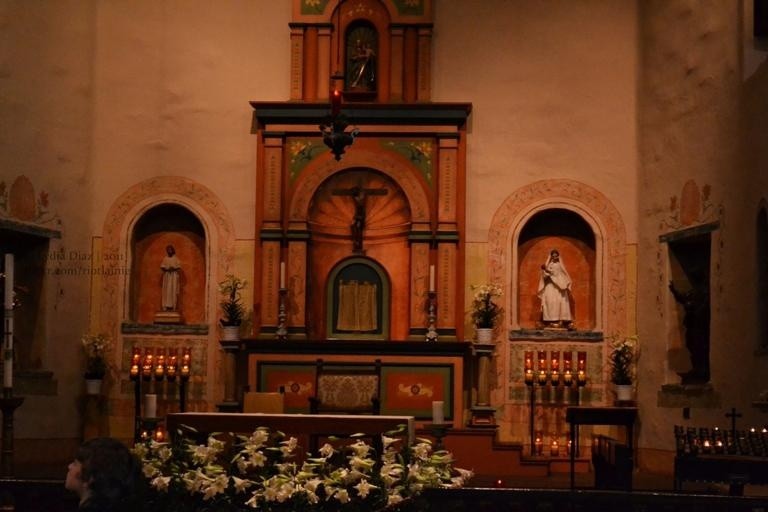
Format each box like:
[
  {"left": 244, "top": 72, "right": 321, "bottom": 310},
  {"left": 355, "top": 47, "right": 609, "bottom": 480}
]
[
  {"left": 537, "top": 250, "right": 573, "bottom": 328},
  {"left": 65, "top": 438, "right": 148, "bottom": 512},
  {"left": 349, "top": 39, "right": 375, "bottom": 88},
  {"left": 160, "top": 245, "right": 181, "bottom": 312}
]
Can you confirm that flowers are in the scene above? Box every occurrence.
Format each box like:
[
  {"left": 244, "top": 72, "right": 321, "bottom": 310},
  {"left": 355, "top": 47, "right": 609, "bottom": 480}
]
[
  {"left": 463, "top": 281, "right": 506, "bottom": 329},
  {"left": 215, "top": 272, "right": 248, "bottom": 326},
  {"left": 81, "top": 328, "right": 115, "bottom": 380},
  {"left": 127, "top": 422, "right": 458, "bottom": 509},
  {"left": 605, "top": 330, "right": 640, "bottom": 384}
]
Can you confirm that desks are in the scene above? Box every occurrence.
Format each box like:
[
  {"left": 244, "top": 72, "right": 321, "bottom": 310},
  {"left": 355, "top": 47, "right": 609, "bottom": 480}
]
[{"left": 565, "top": 405, "right": 641, "bottom": 492}]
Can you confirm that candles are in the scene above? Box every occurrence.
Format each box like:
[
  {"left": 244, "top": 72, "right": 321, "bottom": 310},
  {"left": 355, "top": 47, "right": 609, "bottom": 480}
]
[
  {"left": 431, "top": 398, "right": 446, "bottom": 425},
  {"left": 525, "top": 348, "right": 587, "bottom": 385},
  {"left": 535, "top": 441, "right": 573, "bottom": 456},
  {"left": 144, "top": 392, "right": 158, "bottom": 417},
  {"left": 428, "top": 264, "right": 436, "bottom": 292},
  {"left": 130, "top": 354, "right": 191, "bottom": 378},
  {"left": 280, "top": 261, "right": 286, "bottom": 290}
]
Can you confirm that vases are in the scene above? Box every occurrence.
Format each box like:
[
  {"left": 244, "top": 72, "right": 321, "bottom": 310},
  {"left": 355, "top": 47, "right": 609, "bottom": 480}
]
[
  {"left": 475, "top": 328, "right": 494, "bottom": 345},
  {"left": 85, "top": 379, "right": 103, "bottom": 396},
  {"left": 612, "top": 382, "right": 636, "bottom": 406},
  {"left": 221, "top": 327, "right": 241, "bottom": 341}
]
